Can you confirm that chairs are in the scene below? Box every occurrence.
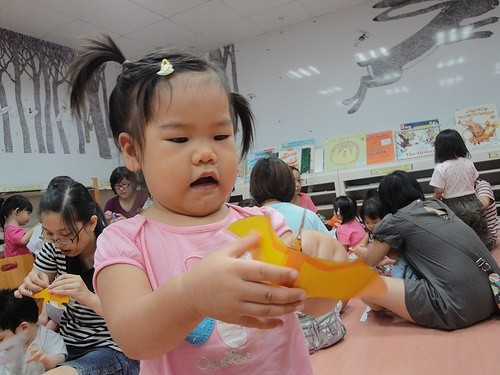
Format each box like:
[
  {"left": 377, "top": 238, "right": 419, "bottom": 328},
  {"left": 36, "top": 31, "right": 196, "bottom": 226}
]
[{"left": 0, "top": 253, "right": 34, "bottom": 292}]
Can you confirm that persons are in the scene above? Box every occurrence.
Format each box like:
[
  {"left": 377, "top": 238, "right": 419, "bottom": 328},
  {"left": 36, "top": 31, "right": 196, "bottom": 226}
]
[
  {"left": 0, "top": 128, "right": 500, "bottom": 375},
  {"left": 70, "top": 33, "right": 347, "bottom": 375}
]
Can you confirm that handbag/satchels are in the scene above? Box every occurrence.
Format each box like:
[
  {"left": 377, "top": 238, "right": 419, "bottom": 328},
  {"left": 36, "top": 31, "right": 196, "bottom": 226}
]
[
  {"left": 297, "top": 308, "right": 347, "bottom": 355},
  {"left": 489, "top": 271, "right": 500, "bottom": 312}
]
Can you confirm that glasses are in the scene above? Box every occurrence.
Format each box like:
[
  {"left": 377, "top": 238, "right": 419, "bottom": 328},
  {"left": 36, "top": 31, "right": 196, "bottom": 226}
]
[
  {"left": 115, "top": 183, "right": 131, "bottom": 188},
  {"left": 295, "top": 179, "right": 304, "bottom": 183},
  {"left": 38, "top": 222, "right": 92, "bottom": 246}
]
